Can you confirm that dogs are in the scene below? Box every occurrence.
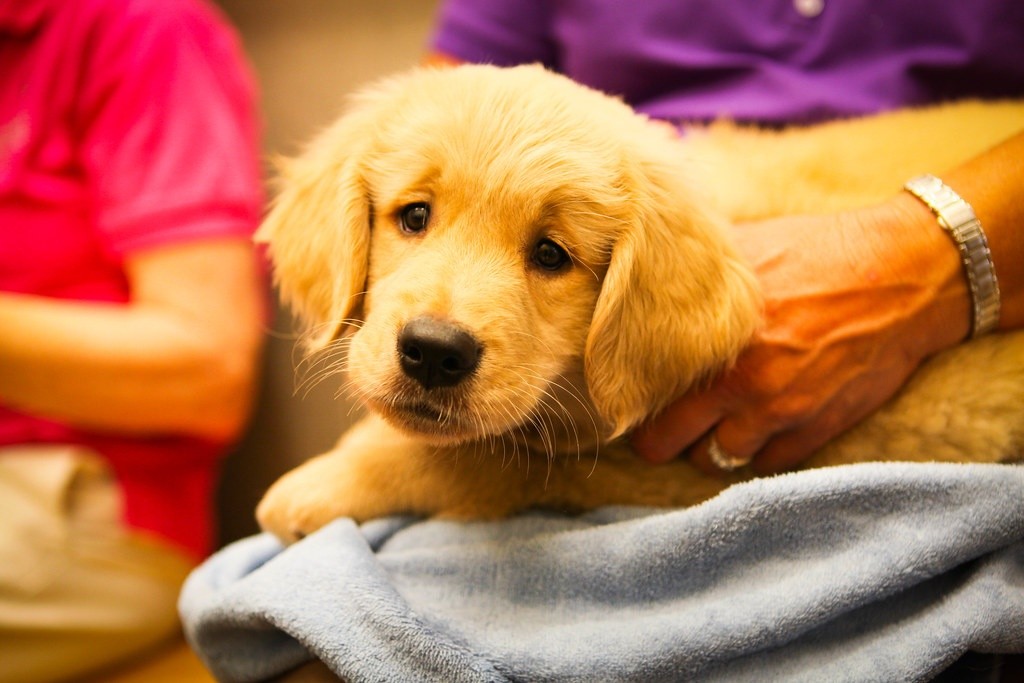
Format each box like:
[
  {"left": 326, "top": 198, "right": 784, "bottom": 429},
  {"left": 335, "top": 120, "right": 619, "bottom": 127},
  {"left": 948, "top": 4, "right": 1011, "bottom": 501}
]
[{"left": 252, "top": 63, "right": 1024, "bottom": 552}]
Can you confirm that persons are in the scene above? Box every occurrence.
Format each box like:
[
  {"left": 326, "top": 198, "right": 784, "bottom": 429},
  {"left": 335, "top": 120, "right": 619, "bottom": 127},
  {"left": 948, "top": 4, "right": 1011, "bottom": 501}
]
[
  {"left": 0, "top": 0, "right": 271, "bottom": 682},
  {"left": 425, "top": 3, "right": 1024, "bottom": 480}
]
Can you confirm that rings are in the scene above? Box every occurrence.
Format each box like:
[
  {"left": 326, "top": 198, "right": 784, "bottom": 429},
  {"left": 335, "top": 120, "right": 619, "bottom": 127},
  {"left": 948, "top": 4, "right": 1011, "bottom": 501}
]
[{"left": 708, "top": 428, "right": 756, "bottom": 472}]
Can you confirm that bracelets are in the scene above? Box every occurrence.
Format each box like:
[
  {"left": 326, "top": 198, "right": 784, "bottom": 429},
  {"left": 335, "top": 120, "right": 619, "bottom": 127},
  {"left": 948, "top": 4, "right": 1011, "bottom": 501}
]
[{"left": 900, "top": 172, "right": 1004, "bottom": 345}]
